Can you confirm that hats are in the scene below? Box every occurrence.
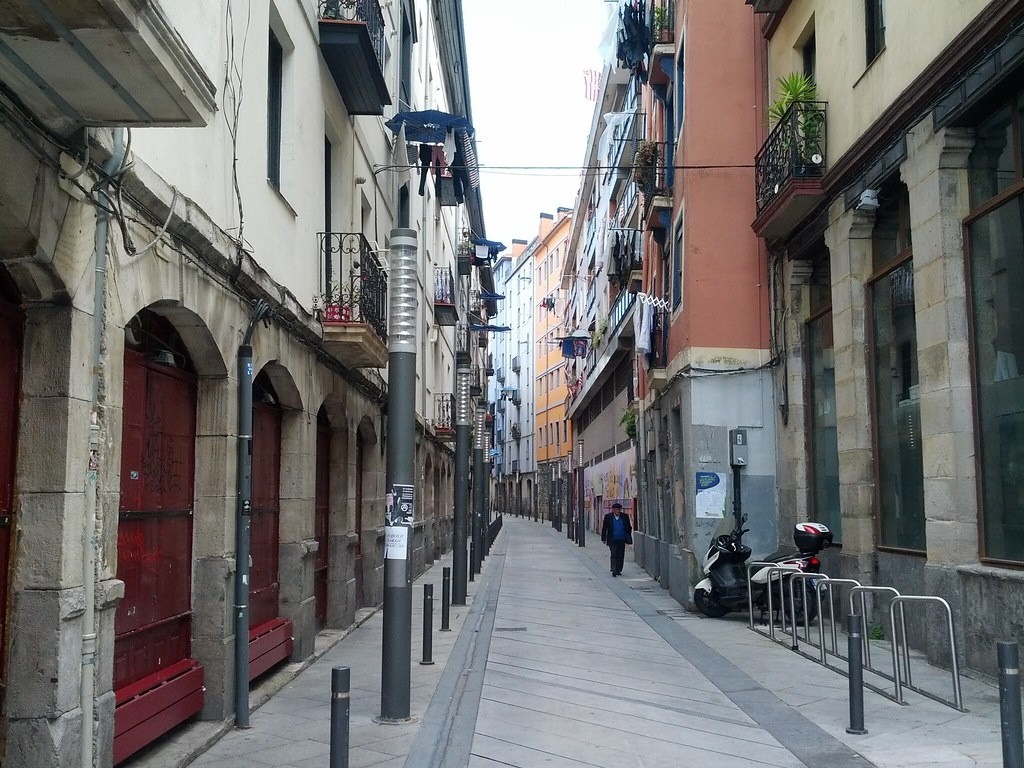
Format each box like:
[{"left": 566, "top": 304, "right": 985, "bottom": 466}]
[{"left": 612, "top": 504, "right": 622, "bottom": 509}]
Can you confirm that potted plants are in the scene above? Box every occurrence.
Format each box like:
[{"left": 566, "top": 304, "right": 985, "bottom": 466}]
[
  {"left": 652, "top": 6, "right": 674, "bottom": 44},
  {"left": 767, "top": 71, "right": 826, "bottom": 176}
]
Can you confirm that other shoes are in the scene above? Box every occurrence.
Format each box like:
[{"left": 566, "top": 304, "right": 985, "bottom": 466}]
[{"left": 613, "top": 571, "right": 621, "bottom": 576}]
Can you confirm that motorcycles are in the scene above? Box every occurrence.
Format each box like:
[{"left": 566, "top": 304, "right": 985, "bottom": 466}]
[{"left": 693, "top": 512, "right": 833, "bottom": 627}]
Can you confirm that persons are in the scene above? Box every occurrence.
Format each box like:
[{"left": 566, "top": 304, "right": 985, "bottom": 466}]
[{"left": 601, "top": 503, "right": 633, "bottom": 577}]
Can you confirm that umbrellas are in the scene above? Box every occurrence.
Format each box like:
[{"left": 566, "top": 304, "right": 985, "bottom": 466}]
[
  {"left": 480, "top": 293, "right": 505, "bottom": 299},
  {"left": 474, "top": 238, "right": 507, "bottom": 251},
  {"left": 473, "top": 323, "right": 512, "bottom": 332},
  {"left": 385, "top": 109, "right": 475, "bottom": 144}
]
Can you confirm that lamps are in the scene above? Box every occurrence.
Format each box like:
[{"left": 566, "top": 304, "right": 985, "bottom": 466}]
[{"left": 856, "top": 186, "right": 883, "bottom": 212}]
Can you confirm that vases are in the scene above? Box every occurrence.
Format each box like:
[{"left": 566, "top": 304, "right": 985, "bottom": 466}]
[{"left": 326, "top": 305, "right": 351, "bottom": 322}]
